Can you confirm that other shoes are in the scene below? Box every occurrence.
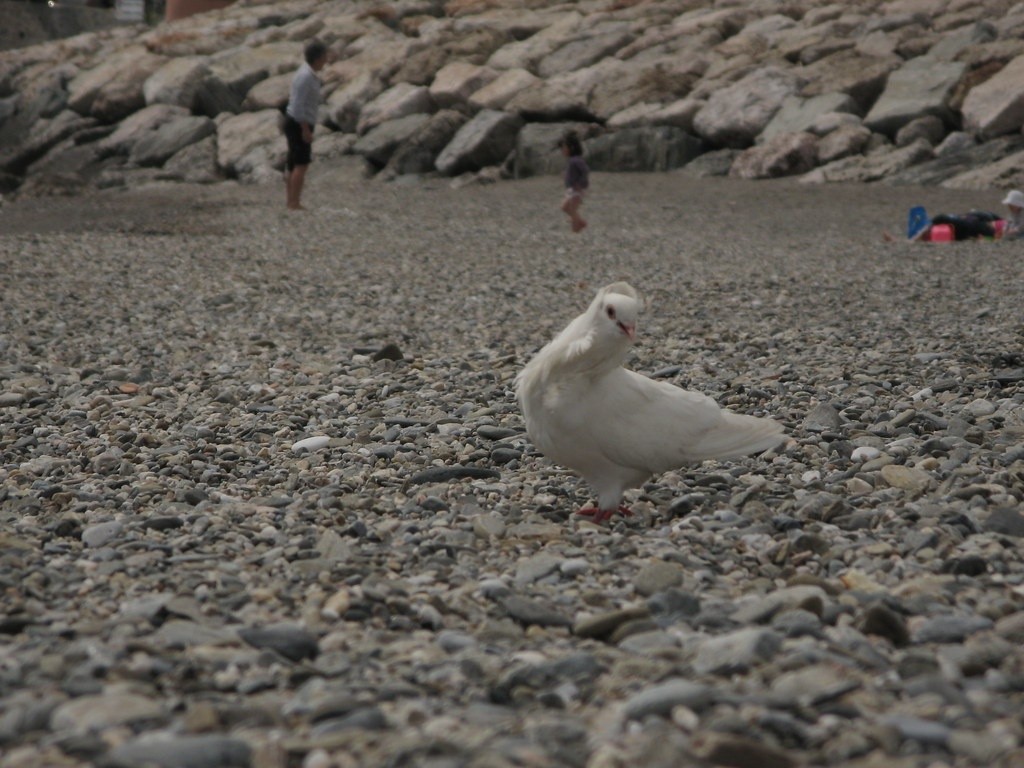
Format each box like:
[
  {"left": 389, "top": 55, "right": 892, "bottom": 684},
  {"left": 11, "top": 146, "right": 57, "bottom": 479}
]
[{"left": 575, "top": 220, "right": 586, "bottom": 231}]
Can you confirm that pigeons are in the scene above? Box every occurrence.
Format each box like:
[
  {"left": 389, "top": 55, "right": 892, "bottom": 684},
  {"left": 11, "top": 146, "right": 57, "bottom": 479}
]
[{"left": 510, "top": 278, "right": 791, "bottom": 525}]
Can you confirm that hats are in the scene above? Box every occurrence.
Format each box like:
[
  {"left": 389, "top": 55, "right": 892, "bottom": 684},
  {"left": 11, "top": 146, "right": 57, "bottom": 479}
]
[{"left": 1001, "top": 190, "right": 1023, "bottom": 208}]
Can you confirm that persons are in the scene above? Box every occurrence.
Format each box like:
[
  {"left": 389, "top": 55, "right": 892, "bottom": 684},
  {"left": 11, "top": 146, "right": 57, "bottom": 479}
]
[
  {"left": 559, "top": 130, "right": 590, "bottom": 232},
  {"left": 280, "top": 38, "right": 327, "bottom": 209},
  {"left": 933, "top": 210, "right": 1005, "bottom": 238},
  {"left": 1003, "top": 191, "right": 1024, "bottom": 239}
]
